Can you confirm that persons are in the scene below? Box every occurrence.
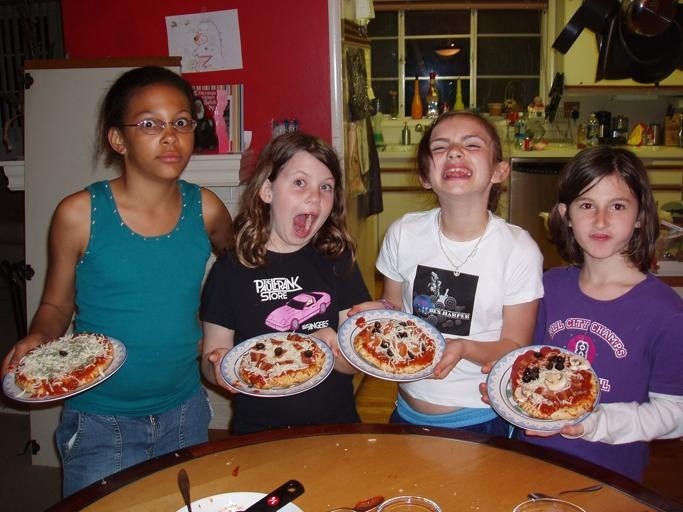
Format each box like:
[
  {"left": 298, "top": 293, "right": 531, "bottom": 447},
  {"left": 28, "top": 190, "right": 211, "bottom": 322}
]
[
  {"left": 1, "top": 66, "right": 236, "bottom": 500},
  {"left": 348, "top": 112, "right": 543, "bottom": 443},
  {"left": 480, "top": 146, "right": 682, "bottom": 484},
  {"left": 198, "top": 131, "right": 375, "bottom": 435}
]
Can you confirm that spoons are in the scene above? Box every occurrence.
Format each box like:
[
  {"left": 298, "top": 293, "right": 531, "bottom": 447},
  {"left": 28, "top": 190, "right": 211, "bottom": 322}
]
[{"left": 525, "top": 483, "right": 604, "bottom": 503}]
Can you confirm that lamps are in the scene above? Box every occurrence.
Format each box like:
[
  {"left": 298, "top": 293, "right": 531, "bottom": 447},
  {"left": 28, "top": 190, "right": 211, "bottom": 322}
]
[{"left": 433, "top": 47, "right": 461, "bottom": 57}]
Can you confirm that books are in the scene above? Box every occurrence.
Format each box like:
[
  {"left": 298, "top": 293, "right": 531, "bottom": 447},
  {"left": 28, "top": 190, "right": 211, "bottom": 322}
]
[{"left": 188, "top": 83, "right": 245, "bottom": 154}]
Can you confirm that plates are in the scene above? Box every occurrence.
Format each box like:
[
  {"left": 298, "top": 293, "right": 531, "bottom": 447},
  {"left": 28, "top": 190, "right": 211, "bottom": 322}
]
[
  {"left": 218, "top": 331, "right": 334, "bottom": 401},
  {"left": 1, "top": 332, "right": 128, "bottom": 404},
  {"left": 335, "top": 308, "right": 448, "bottom": 382},
  {"left": 486, "top": 343, "right": 601, "bottom": 434}
]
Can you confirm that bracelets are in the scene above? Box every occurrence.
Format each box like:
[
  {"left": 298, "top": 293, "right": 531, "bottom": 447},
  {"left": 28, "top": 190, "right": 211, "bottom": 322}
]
[{"left": 378, "top": 298, "right": 395, "bottom": 311}]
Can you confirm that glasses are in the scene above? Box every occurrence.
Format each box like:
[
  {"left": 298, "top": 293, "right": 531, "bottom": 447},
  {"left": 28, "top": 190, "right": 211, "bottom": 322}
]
[{"left": 118, "top": 118, "right": 198, "bottom": 136}]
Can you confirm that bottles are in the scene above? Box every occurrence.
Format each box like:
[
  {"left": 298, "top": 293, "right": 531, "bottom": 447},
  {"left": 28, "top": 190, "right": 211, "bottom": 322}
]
[
  {"left": 425, "top": 72, "right": 439, "bottom": 119},
  {"left": 512, "top": 111, "right": 526, "bottom": 151},
  {"left": 585, "top": 113, "right": 598, "bottom": 147},
  {"left": 410, "top": 72, "right": 423, "bottom": 119}
]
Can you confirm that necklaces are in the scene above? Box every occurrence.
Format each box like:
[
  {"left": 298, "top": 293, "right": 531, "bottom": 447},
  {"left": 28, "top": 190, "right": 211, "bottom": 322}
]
[{"left": 437, "top": 211, "right": 489, "bottom": 276}]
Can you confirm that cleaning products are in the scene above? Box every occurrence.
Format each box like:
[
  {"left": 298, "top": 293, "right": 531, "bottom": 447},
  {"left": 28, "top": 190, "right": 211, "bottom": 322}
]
[{"left": 402, "top": 121, "right": 410, "bottom": 145}]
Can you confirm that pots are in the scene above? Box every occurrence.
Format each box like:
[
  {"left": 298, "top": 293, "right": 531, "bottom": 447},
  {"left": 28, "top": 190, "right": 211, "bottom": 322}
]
[{"left": 553, "top": 0, "right": 682, "bottom": 85}]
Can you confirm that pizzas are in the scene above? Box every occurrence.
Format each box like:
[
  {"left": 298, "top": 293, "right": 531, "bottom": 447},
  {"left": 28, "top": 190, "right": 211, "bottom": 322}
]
[
  {"left": 11, "top": 332, "right": 114, "bottom": 395},
  {"left": 239, "top": 334, "right": 328, "bottom": 391},
  {"left": 513, "top": 346, "right": 597, "bottom": 419},
  {"left": 352, "top": 316, "right": 435, "bottom": 375}
]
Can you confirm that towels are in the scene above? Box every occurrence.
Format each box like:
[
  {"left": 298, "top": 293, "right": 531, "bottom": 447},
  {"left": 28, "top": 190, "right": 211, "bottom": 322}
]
[{"left": 347, "top": 116, "right": 384, "bottom": 219}]
